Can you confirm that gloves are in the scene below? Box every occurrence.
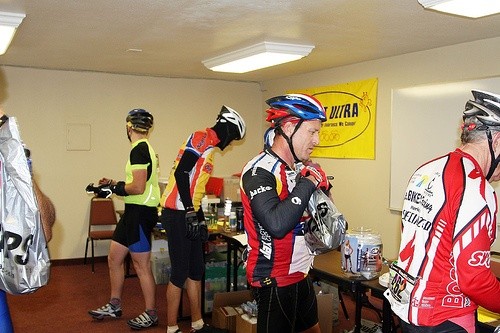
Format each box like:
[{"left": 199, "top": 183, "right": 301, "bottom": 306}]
[
  {"left": 184, "top": 208, "right": 208, "bottom": 242},
  {"left": 300, "top": 163, "right": 330, "bottom": 191}
]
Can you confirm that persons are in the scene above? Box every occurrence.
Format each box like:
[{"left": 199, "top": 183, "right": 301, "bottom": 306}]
[
  {"left": 160, "top": 105, "right": 246, "bottom": 333},
  {"left": 0, "top": 64, "right": 55, "bottom": 333},
  {"left": 240, "top": 94, "right": 333, "bottom": 333},
  {"left": 382, "top": 88, "right": 500, "bottom": 333},
  {"left": 87, "top": 108, "right": 160, "bottom": 329}
]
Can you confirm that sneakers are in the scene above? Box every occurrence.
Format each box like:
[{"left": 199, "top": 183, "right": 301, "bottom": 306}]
[
  {"left": 87, "top": 303, "right": 123, "bottom": 320},
  {"left": 127, "top": 309, "right": 160, "bottom": 330}
]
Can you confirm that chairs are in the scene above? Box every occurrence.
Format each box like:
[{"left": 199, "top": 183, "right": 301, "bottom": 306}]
[
  {"left": 84, "top": 195, "right": 118, "bottom": 275},
  {"left": 338, "top": 280, "right": 401, "bottom": 333}
]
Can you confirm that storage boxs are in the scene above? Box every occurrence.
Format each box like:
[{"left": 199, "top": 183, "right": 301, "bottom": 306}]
[
  {"left": 212, "top": 287, "right": 333, "bottom": 333},
  {"left": 219, "top": 176, "right": 241, "bottom": 203},
  {"left": 151, "top": 251, "right": 172, "bottom": 285}
]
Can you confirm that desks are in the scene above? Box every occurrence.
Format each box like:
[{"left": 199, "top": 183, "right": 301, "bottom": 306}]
[
  {"left": 220, "top": 231, "right": 390, "bottom": 333},
  {"left": 360, "top": 279, "right": 500, "bottom": 333},
  {"left": 117, "top": 209, "right": 230, "bottom": 278}
]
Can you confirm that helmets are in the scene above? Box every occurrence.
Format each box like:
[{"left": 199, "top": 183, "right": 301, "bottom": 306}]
[
  {"left": 462, "top": 89, "right": 500, "bottom": 131},
  {"left": 264, "top": 92, "right": 327, "bottom": 129},
  {"left": 216, "top": 104, "right": 246, "bottom": 141},
  {"left": 125, "top": 108, "right": 154, "bottom": 132}
]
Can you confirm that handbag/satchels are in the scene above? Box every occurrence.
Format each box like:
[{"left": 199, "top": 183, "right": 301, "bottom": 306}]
[
  {"left": 0, "top": 116, "right": 52, "bottom": 296},
  {"left": 303, "top": 188, "right": 348, "bottom": 256}
]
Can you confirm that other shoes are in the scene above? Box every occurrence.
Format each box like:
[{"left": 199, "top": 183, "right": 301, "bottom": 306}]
[{"left": 173, "top": 323, "right": 234, "bottom": 333}]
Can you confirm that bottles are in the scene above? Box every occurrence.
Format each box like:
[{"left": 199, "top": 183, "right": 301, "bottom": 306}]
[
  {"left": 236, "top": 207, "right": 244, "bottom": 232},
  {"left": 341, "top": 227, "right": 384, "bottom": 281},
  {"left": 229, "top": 212, "right": 237, "bottom": 228},
  {"left": 204, "top": 203, "right": 218, "bottom": 230}
]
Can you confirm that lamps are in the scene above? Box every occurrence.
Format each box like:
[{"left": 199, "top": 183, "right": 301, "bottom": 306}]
[
  {"left": 200, "top": 41, "right": 315, "bottom": 73},
  {"left": 417, "top": 0, "right": 500, "bottom": 19},
  {"left": 0, "top": 11, "right": 27, "bottom": 55}
]
